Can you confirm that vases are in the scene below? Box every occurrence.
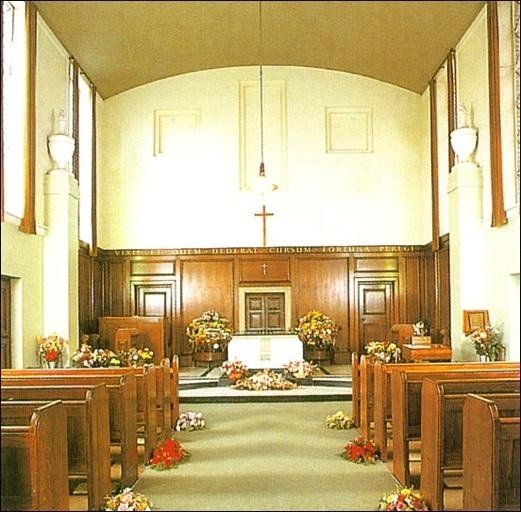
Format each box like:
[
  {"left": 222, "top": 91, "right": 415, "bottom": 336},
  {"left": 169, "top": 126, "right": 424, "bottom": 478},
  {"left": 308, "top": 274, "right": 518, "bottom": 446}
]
[
  {"left": 192, "top": 346, "right": 226, "bottom": 370},
  {"left": 305, "top": 340, "right": 335, "bottom": 363}
]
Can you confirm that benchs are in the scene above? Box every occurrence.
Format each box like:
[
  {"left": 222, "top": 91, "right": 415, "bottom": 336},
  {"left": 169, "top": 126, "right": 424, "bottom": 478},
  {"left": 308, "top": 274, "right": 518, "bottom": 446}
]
[
  {"left": 347, "top": 346, "right": 521, "bottom": 510},
  {"left": 2, "top": 340, "right": 186, "bottom": 511}
]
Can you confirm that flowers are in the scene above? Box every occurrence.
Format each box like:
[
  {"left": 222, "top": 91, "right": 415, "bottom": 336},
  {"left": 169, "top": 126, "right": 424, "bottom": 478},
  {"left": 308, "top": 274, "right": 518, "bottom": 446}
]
[
  {"left": 468, "top": 324, "right": 501, "bottom": 349},
  {"left": 364, "top": 340, "right": 396, "bottom": 357},
  {"left": 189, "top": 308, "right": 229, "bottom": 351},
  {"left": 179, "top": 408, "right": 204, "bottom": 430},
  {"left": 36, "top": 335, "right": 65, "bottom": 363},
  {"left": 338, "top": 437, "right": 378, "bottom": 462},
  {"left": 218, "top": 360, "right": 318, "bottom": 391},
  {"left": 327, "top": 412, "right": 353, "bottom": 430},
  {"left": 297, "top": 309, "right": 338, "bottom": 346}
]
